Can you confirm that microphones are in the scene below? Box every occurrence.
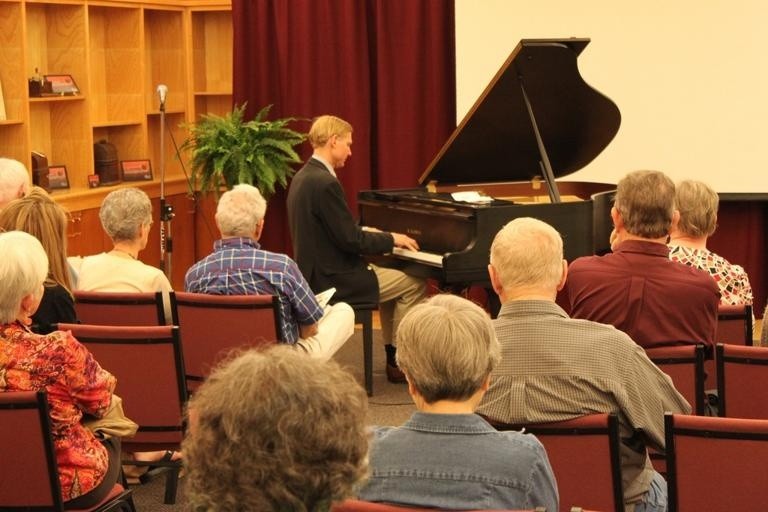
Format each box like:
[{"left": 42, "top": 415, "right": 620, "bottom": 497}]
[{"left": 156, "top": 84, "right": 168, "bottom": 108}]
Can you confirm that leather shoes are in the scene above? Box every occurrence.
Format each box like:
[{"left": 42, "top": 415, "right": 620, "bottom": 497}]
[{"left": 386, "top": 363, "right": 407, "bottom": 384}]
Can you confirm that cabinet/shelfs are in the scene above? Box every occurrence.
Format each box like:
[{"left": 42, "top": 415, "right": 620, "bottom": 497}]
[
  {"left": 0, "top": 0, "right": 236, "bottom": 302},
  {"left": 101, "top": 193, "right": 195, "bottom": 295},
  {"left": 65, "top": 208, "right": 101, "bottom": 257},
  {"left": 195, "top": 191, "right": 224, "bottom": 265}
]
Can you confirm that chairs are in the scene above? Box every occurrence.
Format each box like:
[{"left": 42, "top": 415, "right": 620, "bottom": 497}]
[
  {"left": 706, "top": 304, "right": 754, "bottom": 395},
  {"left": 48, "top": 321, "right": 189, "bottom": 504},
  {"left": 328, "top": 498, "right": 545, "bottom": 512},
  {"left": 68, "top": 290, "right": 165, "bottom": 326},
  {"left": 30, "top": 322, "right": 41, "bottom": 335},
  {"left": 715, "top": 341, "right": 768, "bottom": 419},
  {"left": 644, "top": 343, "right": 705, "bottom": 476},
  {"left": 487, "top": 414, "right": 621, "bottom": 512},
  {"left": 0, "top": 389, "right": 134, "bottom": 512},
  {"left": 666, "top": 412, "right": 767, "bottom": 512},
  {"left": 570, "top": 503, "right": 596, "bottom": 509}
]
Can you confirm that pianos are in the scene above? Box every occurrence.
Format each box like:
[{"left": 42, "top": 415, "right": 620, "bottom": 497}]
[{"left": 356, "top": 37, "right": 623, "bottom": 318}]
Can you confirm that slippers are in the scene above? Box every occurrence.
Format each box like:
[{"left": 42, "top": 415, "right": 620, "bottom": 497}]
[{"left": 139, "top": 449, "right": 188, "bottom": 484}]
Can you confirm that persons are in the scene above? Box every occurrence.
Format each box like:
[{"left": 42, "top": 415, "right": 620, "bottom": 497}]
[
  {"left": 180, "top": 339, "right": 374, "bottom": 512},
  {"left": 1, "top": 187, "right": 83, "bottom": 323},
  {"left": 354, "top": 294, "right": 560, "bottom": 512},
  {"left": 666, "top": 179, "right": 755, "bottom": 334},
  {"left": 66, "top": 188, "right": 185, "bottom": 483},
  {"left": 475, "top": 217, "right": 692, "bottom": 512},
  {"left": 555, "top": 170, "right": 718, "bottom": 418},
  {"left": 184, "top": 184, "right": 354, "bottom": 363},
  {"left": 0, "top": 231, "right": 138, "bottom": 512},
  {"left": 287, "top": 115, "right": 428, "bottom": 384},
  {"left": 1, "top": 157, "right": 31, "bottom": 207}
]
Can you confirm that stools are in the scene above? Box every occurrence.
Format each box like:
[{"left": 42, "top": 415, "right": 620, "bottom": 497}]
[
  {"left": 349, "top": 301, "right": 380, "bottom": 397},
  {"left": 169, "top": 288, "right": 282, "bottom": 404}
]
